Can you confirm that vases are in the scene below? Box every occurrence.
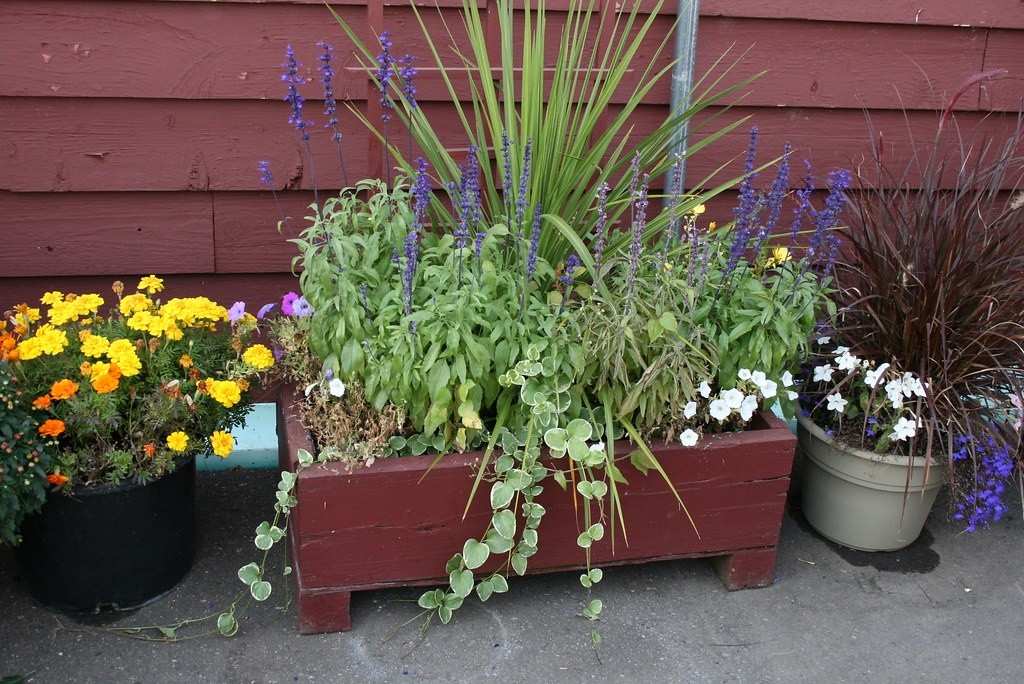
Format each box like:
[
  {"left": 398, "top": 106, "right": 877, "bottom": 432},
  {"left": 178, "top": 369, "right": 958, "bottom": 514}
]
[
  {"left": 14, "top": 453, "right": 198, "bottom": 616},
  {"left": 277, "top": 377, "right": 798, "bottom": 636}
]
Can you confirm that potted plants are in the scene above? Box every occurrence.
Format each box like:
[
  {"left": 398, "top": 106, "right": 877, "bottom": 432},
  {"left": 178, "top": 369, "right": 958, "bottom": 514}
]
[{"left": 796, "top": 61, "right": 1023, "bottom": 552}]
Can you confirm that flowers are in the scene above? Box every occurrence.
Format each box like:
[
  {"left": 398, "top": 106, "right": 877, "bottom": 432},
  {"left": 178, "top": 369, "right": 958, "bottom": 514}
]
[
  {"left": 0, "top": 274, "right": 275, "bottom": 550},
  {"left": 57, "top": 0, "right": 852, "bottom": 666},
  {"left": 802, "top": 328, "right": 1023, "bottom": 533}
]
